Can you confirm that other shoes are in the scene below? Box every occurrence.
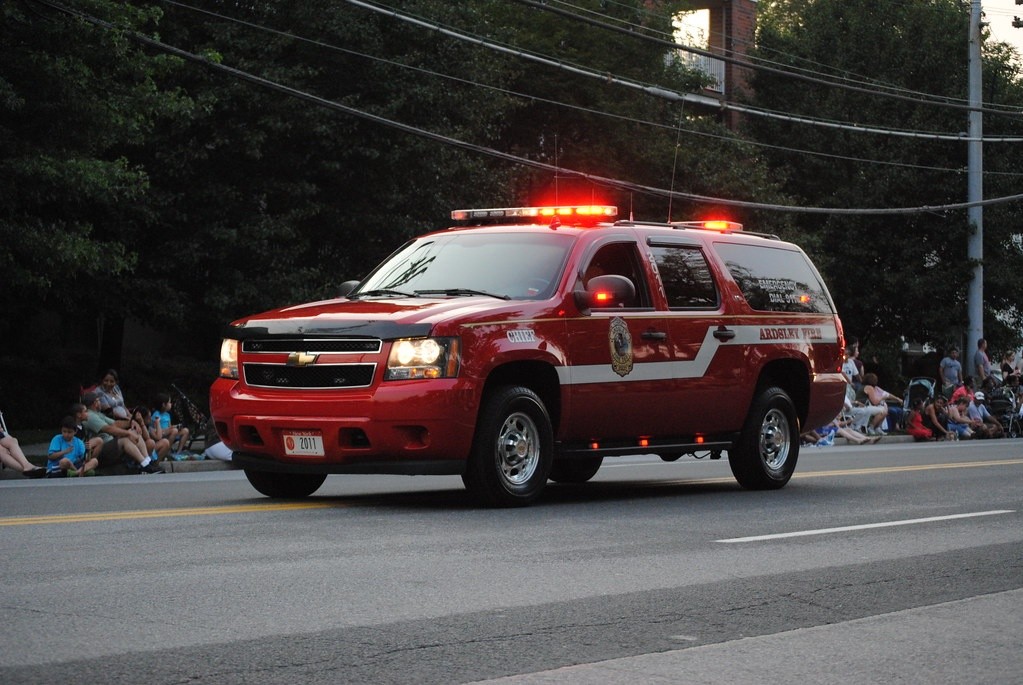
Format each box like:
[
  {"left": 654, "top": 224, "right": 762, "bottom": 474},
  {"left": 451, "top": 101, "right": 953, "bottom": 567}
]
[
  {"left": 858, "top": 438, "right": 870, "bottom": 445},
  {"left": 969, "top": 431, "right": 979, "bottom": 439},
  {"left": 867, "top": 427, "right": 875, "bottom": 435},
  {"left": 871, "top": 436, "right": 882, "bottom": 444},
  {"left": 876, "top": 427, "right": 888, "bottom": 435}
]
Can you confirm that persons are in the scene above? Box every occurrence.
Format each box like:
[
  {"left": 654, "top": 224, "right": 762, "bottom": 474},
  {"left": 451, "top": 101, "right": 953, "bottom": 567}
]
[
  {"left": 0, "top": 369, "right": 190, "bottom": 478},
  {"left": 803, "top": 335, "right": 1023, "bottom": 444}
]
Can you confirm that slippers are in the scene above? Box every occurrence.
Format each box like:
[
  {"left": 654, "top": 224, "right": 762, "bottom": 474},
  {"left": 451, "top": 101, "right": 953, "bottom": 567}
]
[{"left": 22, "top": 466, "right": 47, "bottom": 477}]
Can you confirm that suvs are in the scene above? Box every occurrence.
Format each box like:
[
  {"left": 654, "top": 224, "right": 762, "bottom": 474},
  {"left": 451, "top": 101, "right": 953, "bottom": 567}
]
[{"left": 210, "top": 201, "right": 851, "bottom": 512}]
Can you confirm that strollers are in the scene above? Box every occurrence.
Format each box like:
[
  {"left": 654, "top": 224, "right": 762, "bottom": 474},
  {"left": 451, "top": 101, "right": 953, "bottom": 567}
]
[
  {"left": 987, "top": 386, "right": 1021, "bottom": 438},
  {"left": 898, "top": 376, "right": 938, "bottom": 431}
]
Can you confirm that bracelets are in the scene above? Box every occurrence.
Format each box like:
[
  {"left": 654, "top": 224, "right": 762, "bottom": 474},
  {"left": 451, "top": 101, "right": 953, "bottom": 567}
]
[{"left": 141, "top": 423, "right": 145, "bottom": 426}]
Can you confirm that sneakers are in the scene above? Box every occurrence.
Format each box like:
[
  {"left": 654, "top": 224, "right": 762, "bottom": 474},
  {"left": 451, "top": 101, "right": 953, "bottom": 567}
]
[
  {"left": 67, "top": 469, "right": 76, "bottom": 477},
  {"left": 826, "top": 429, "right": 835, "bottom": 442},
  {"left": 140, "top": 459, "right": 163, "bottom": 475},
  {"left": 817, "top": 440, "right": 834, "bottom": 447},
  {"left": 84, "top": 469, "right": 95, "bottom": 477}
]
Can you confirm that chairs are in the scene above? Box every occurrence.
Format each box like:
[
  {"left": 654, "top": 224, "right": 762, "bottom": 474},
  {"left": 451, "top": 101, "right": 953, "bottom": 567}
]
[{"left": 842, "top": 384, "right": 868, "bottom": 434}]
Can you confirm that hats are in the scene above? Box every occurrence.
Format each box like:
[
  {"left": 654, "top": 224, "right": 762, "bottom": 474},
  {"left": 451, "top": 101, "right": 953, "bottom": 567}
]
[{"left": 974, "top": 391, "right": 985, "bottom": 400}]
